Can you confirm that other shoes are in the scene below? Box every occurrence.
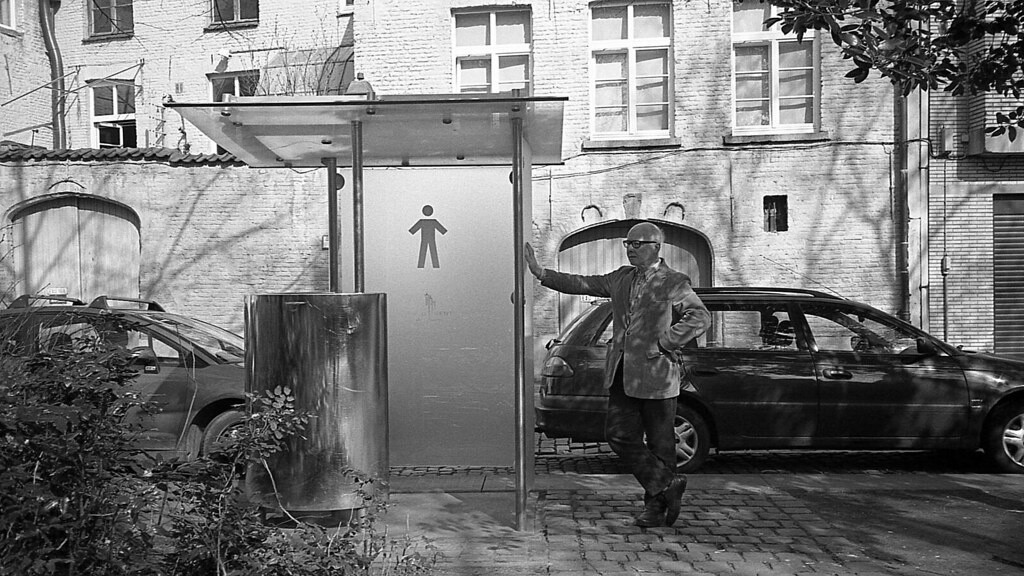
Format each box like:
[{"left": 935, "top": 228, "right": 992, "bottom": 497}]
[
  {"left": 656, "top": 475, "right": 688, "bottom": 527},
  {"left": 637, "top": 493, "right": 666, "bottom": 527}
]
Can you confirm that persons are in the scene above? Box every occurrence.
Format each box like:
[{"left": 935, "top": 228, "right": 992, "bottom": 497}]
[{"left": 525, "top": 222, "right": 712, "bottom": 526}]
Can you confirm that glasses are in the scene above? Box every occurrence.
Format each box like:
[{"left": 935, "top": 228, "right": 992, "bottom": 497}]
[{"left": 623, "top": 241, "right": 657, "bottom": 248}]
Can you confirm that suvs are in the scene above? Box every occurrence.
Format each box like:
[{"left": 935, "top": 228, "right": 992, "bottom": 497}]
[
  {"left": 534, "top": 285, "right": 1024, "bottom": 476},
  {"left": 0, "top": 293, "right": 251, "bottom": 472}
]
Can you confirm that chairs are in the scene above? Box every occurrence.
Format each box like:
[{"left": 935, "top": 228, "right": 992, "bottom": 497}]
[{"left": 758, "top": 316, "right": 794, "bottom": 350}]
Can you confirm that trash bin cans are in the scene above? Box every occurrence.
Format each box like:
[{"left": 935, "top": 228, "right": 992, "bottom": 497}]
[{"left": 245, "top": 290, "right": 389, "bottom": 512}]
